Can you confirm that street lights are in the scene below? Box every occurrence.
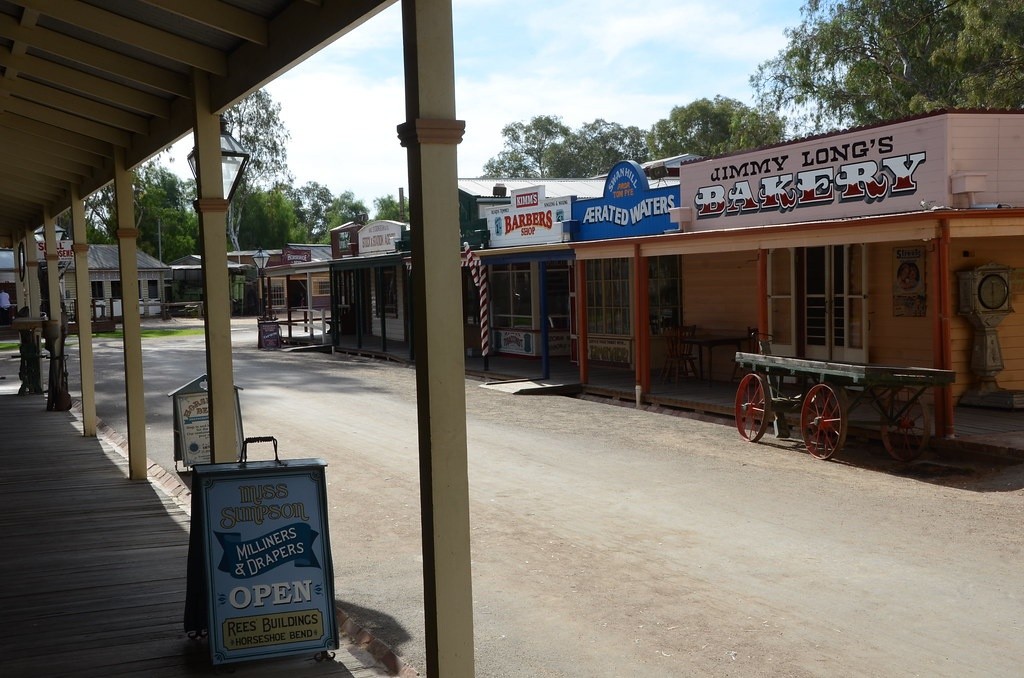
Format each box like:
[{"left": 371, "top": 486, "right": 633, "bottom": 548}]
[{"left": 253, "top": 248, "right": 270, "bottom": 316}]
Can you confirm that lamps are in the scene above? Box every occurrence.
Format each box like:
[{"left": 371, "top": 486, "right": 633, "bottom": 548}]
[
  {"left": 648, "top": 162, "right": 669, "bottom": 187},
  {"left": 493, "top": 184, "right": 507, "bottom": 198},
  {"left": 354, "top": 213, "right": 366, "bottom": 226}
]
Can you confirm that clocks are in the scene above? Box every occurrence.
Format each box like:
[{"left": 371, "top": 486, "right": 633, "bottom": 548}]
[{"left": 977, "top": 274, "right": 1008, "bottom": 310}]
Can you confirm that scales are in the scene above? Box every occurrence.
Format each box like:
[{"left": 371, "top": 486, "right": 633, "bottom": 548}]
[{"left": 958, "top": 262, "right": 1024, "bottom": 410}]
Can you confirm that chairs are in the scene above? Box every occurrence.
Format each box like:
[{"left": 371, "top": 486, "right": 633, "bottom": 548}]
[
  {"left": 660, "top": 315, "right": 699, "bottom": 386},
  {"left": 729, "top": 326, "right": 759, "bottom": 384}
]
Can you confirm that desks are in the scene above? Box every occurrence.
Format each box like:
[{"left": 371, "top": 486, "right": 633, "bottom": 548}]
[{"left": 671, "top": 333, "right": 748, "bottom": 388}]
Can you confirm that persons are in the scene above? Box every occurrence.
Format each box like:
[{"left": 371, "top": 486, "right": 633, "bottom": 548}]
[{"left": 0, "top": 290, "right": 11, "bottom": 309}]
